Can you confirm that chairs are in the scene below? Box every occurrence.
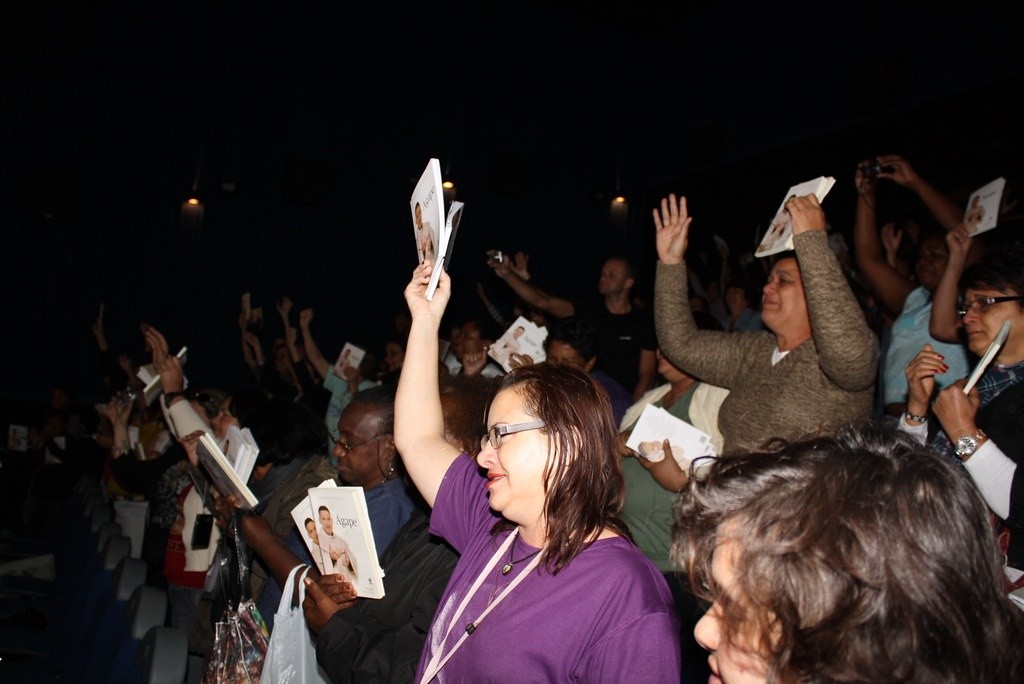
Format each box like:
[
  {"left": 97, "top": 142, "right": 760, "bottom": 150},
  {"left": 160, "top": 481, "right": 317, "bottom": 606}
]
[{"left": 0, "top": 487, "right": 189, "bottom": 684}]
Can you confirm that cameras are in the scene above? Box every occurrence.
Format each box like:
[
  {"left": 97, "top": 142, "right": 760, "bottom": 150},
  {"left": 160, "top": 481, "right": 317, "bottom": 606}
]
[
  {"left": 494, "top": 251, "right": 503, "bottom": 263},
  {"left": 191, "top": 514, "right": 213, "bottom": 549}
]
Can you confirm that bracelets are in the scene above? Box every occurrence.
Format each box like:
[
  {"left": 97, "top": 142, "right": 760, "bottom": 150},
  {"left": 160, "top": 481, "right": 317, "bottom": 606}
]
[
  {"left": 905, "top": 412, "right": 926, "bottom": 423},
  {"left": 165, "top": 391, "right": 184, "bottom": 408}
]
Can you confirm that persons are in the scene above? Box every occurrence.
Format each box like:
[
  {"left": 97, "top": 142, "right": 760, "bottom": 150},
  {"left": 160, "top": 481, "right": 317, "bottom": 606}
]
[
  {"left": 335, "top": 349, "right": 350, "bottom": 377},
  {"left": 496, "top": 325, "right": 524, "bottom": 361},
  {"left": 305, "top": 518, "right": 325, "bottom": 575},
  {"left": 638, "top": 441, "right": 690, "bottom": 470},
  {"left": 414, "top": 202, "right": 436, "bottom": 262},
  {"left": 318, "top": 506, "right": 358, "bottom": 585},
  {"left": 12, "top": 156, "right": 1024, "bottom": 684},
  {"left": 759, "top": 194, "right": 796, "bottom": 252},
  {"left": 965, "top": 195, "right": 985, "bottom": 234}
]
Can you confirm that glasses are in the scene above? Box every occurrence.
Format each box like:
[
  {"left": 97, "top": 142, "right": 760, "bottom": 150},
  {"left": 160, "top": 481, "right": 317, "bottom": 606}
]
[
  {"left": 328, "top": 428, "right": 394, "bottom": 454},
  {"left": 956, "top": 296, "right": 1024, "bottom": 320},
  {"left": 191, "top": 392, "right": 218, "bottom": 411},
  {"left": 480, "top": 420, "right": 547, "bottom": 451}
]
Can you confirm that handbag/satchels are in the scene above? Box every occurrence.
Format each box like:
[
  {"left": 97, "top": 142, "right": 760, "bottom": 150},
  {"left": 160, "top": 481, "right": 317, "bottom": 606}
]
[
  {"left": 200, "top": 505, "right": 271, "bottom": 684},
  {"left": 259, "top": 564, "right": 333, "bottom": 684}
]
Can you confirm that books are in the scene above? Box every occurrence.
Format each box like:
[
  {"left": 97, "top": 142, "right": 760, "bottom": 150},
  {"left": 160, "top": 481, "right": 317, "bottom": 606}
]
[
  {"left": 138, "top": 346, "right": 189, "bottom": 406},
  {"left": 625, "top": 403, "right": 717, "bottom": 472},
  {"left": 331, "top": 342, "right": 365, "bottom": 381},
  {"left": 196, "top": 426, "right": 260, "bottom": 511},
  {"left": 290, "top": 478, "right": 386, "bottom": 580},
  {"left": 754, "top": 176, "right": 836, "bottom": 257},
  {"left": 962, "top": 177, "right": 1006, "bottom": 237},
  {"left": 487, "top": 315, "right": 549, "bottom": 374},
  {"left": 308, "top": 487, "right": 385, "bottom": 600},
  {"left": 963, "top": 320, "right": 1012, "bottom": 395},
  {"left": 409, "top": 158, "right": 465, "bottom": 300}
]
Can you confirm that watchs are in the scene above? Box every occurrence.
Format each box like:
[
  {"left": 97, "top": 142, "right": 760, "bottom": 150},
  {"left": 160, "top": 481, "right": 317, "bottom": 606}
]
[{"left": 955, "top": 430, "right": 986, "bottom": 458}]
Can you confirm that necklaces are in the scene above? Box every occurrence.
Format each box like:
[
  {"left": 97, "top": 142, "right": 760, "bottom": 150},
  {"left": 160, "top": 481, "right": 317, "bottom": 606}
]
[{"left": 502, "top": 532, "right": 543, "bottom": 575}]
